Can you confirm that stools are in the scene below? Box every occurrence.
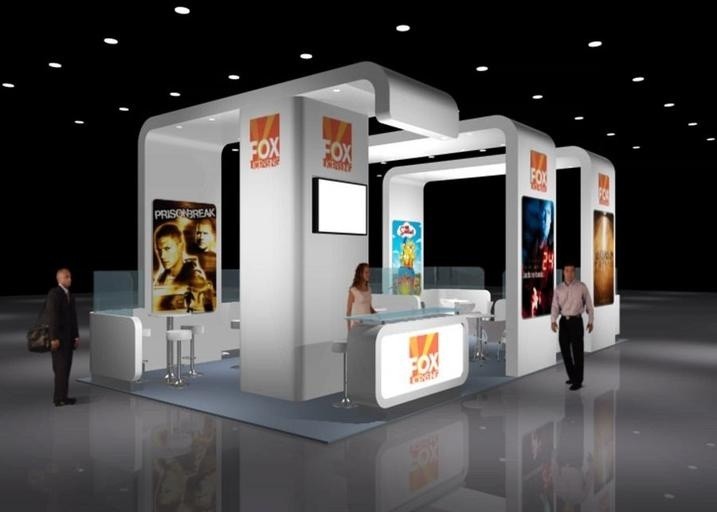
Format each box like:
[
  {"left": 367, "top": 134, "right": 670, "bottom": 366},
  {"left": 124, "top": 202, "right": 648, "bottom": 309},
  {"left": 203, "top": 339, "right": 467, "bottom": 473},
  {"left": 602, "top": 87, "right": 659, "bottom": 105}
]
[{"left": 165, "top": 324, "right": 205, "bottom": 388}]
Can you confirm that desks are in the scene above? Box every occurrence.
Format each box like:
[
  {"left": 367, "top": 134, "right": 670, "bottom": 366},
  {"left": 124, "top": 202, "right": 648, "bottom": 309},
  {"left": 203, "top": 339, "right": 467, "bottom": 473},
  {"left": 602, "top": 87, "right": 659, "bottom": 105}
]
[
  {"left": 148, "top": 311, "right": 191, "bottom": 381},
  {"left": 464, "top": 313, "right": 497, "bottom": 362}
]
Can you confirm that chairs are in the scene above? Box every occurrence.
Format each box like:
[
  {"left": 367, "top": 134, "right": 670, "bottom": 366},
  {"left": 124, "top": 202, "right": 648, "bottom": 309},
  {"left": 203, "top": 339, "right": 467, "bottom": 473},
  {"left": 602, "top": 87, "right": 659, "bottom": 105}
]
[{"left": 484, "top": 298, "right": 509, "bottom": 361}]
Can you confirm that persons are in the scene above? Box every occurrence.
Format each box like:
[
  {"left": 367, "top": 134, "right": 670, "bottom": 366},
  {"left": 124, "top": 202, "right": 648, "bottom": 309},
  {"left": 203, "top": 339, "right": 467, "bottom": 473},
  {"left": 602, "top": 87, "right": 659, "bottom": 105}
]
[
  {"left": 153, "top": 218, "right": 216, "bottom": 313},
  {"left": 550, "top": 264, "right": 594, "bottom": 390},
  {"left": 46, "top": 267, "right": 80, "bottom": 406},
  {"left": 346, "top": 263, "right": 376, "bottom": 333}
]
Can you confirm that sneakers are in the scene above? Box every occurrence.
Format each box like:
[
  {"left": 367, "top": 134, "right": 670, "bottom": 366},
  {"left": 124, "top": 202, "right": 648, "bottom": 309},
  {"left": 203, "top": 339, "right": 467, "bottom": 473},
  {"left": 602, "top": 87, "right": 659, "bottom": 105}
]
[
  {"left": 565, "top": 378, "right": 583, "bottom": 392},
  {"left": 53, "top": 397, "right": 77, "bottom": 407}
]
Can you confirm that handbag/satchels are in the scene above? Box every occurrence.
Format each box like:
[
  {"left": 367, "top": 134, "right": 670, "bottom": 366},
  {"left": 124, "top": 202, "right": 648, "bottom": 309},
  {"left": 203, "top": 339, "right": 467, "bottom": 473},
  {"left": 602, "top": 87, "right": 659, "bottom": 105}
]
[{"left": 25, "top": 291, "right": 52, "bottom": 353}]
